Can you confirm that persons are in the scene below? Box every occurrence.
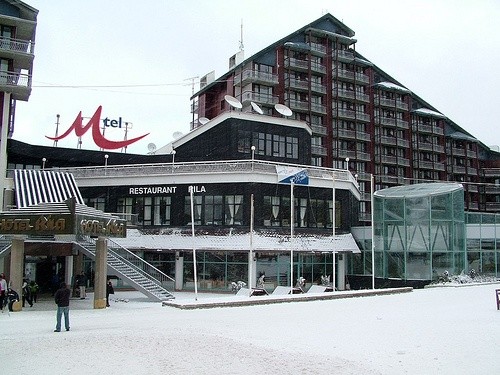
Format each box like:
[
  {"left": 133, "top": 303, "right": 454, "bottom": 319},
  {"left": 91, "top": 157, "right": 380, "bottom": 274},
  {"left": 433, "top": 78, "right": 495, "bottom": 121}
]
[
  {"left": 0, "top": 273, "right": 8, "bottom": 310},
  {"left": 256, "top": 273, "right": 271, "bottom": 296},
  {"left": 106, "top": 277, "right": 114, "bottom": 307},
  {"left": 8, "top": 288, "right": 19, "bottom": 311},
  {"left": 78, "top": 271, "right": 87, "bottom": 299},
  {"left": 54, "top": 283, "right": 70, "bottom": 332},
  {"left": 29, "top": 281, "right": 39, "bottom": 303},
  {"left": 22, "top": 281, "right": 33, "bottom": 307}
]
